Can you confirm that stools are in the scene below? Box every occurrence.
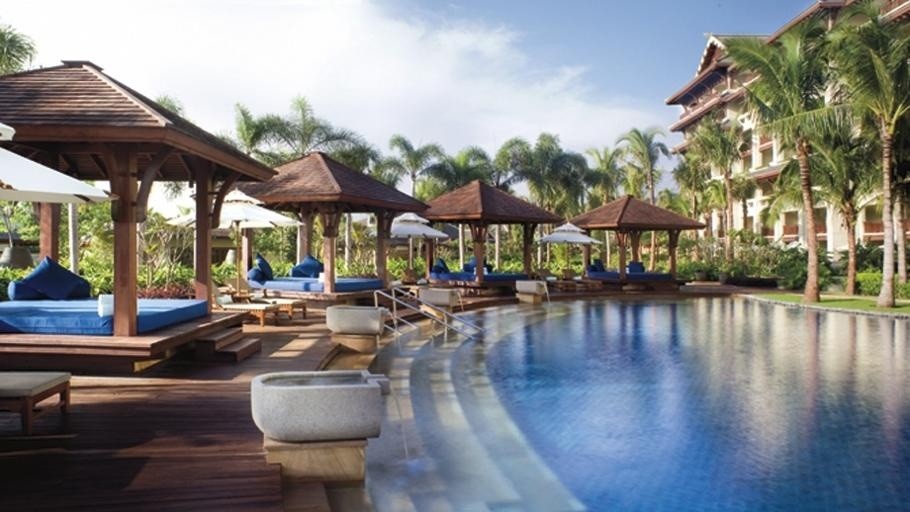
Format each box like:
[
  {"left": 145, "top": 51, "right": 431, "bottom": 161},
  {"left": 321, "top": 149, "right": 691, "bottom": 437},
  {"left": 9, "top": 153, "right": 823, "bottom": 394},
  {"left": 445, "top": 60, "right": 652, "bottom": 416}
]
[{"left": 1, "top": 371, "right": 71, "bottom": 433}]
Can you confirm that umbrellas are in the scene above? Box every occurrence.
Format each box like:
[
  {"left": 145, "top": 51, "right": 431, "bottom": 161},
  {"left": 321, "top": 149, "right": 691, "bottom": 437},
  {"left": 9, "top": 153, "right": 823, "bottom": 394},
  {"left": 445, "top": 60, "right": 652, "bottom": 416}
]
[
  {"left": 533, "top": 219, "right": 604, "bottom": 270},
  {"left": 367, "top": 211, "right": 449, "bottom": 273},
  {"left": 95, "top": 180, "right": 196, "bottom": 276},
  {"left": 0, "top": 122, "right": 122, "bottom": 207},
  {"left": 164, "top": 183, "right": 305, "bottom": 296}
]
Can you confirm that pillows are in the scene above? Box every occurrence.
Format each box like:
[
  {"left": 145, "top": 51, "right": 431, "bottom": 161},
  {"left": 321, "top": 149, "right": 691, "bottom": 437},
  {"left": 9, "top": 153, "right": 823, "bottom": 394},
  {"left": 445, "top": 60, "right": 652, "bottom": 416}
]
[
  {"left": 290, "top": 268, "right": 307, "bottom": 277},
  {"left": 248, "top": 268, "right": 262, "bottom": 281},
  {"left": 256, "top": 252, "right": 273, "bottom": 280},
  {"left": 22, "top": 258, "right": 85, "bottom": 300},
  {"left": 296, "top": 254, "right": 323, "bottom": 278},
  {"left": 628, "top": 260, "right": 645, "bottom": 273},
  {"left": 7, "top": 280, "right": 90, "bottom": 300},
  {"left": 463, "top": 256, "right": 492, "bottom": 274},
  {"left": 430, "top": 258, "right": 448, "bottom": 272},
  {"left": 587, "top": 259, "right": 604, "bottom": 272}
]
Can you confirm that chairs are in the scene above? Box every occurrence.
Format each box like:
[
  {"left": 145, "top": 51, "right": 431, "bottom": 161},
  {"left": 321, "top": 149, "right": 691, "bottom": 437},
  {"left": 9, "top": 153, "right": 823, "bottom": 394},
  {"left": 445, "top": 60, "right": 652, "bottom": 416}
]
[
  {"left": 537, "top": 267, "right": 603, "bottom": 292},
  {"left": 191, "top": 279, "right": 306, "bottom": 326}
]
